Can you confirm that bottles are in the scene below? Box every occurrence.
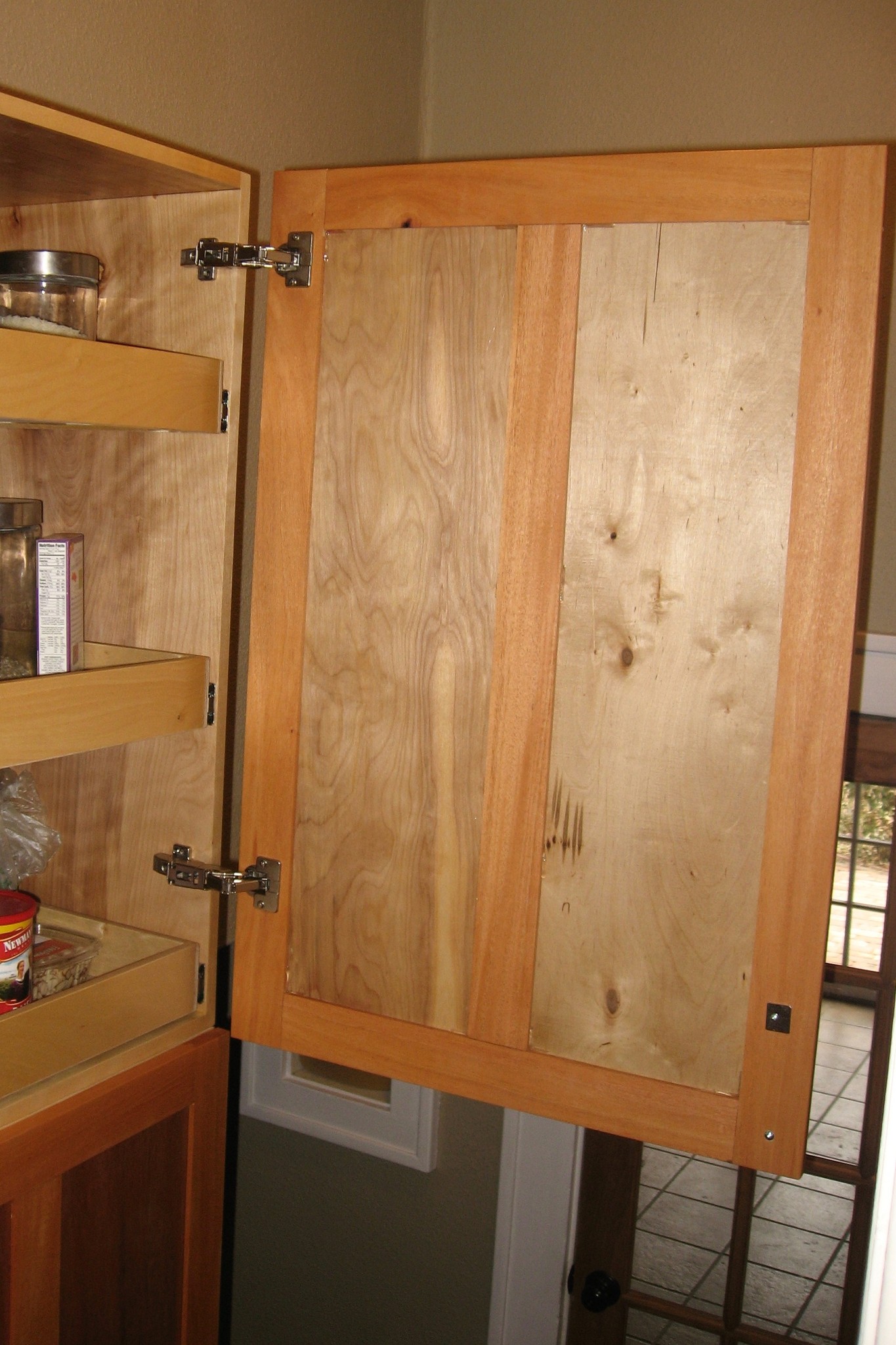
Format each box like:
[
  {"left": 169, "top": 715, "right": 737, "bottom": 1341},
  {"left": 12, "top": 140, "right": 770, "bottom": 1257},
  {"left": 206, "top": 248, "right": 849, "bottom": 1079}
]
[{"left": 0, "top": 497, "right": 43, "bottom": 681}]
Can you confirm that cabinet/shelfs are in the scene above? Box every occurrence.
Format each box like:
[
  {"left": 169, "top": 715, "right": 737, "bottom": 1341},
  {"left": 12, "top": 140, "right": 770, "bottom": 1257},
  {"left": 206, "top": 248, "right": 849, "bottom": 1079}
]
[{"left": 2, "top": 78, "right": 896, "bottom": 1344}]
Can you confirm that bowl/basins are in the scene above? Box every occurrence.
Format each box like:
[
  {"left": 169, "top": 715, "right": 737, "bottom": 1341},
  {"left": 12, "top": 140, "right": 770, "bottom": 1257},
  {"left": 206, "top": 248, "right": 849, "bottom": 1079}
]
[
  {"left": 1, "top": 248, "right": 100, "bottom": 341},
  {"left": 31, "top": 924, "right": 102, "bottom": 1002}
]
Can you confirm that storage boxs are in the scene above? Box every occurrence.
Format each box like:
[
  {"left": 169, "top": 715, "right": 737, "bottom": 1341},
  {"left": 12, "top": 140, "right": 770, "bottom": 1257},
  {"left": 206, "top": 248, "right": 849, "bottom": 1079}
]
[{"left": 34, "top": 530, "right": 90, "bottom": 677}]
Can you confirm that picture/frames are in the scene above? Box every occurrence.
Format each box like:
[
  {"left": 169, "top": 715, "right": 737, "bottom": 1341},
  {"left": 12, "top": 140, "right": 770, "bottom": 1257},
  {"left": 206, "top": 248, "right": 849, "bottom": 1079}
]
[{"left": 231, "top": 1030, "right": 443, "bottom": 1172}]
